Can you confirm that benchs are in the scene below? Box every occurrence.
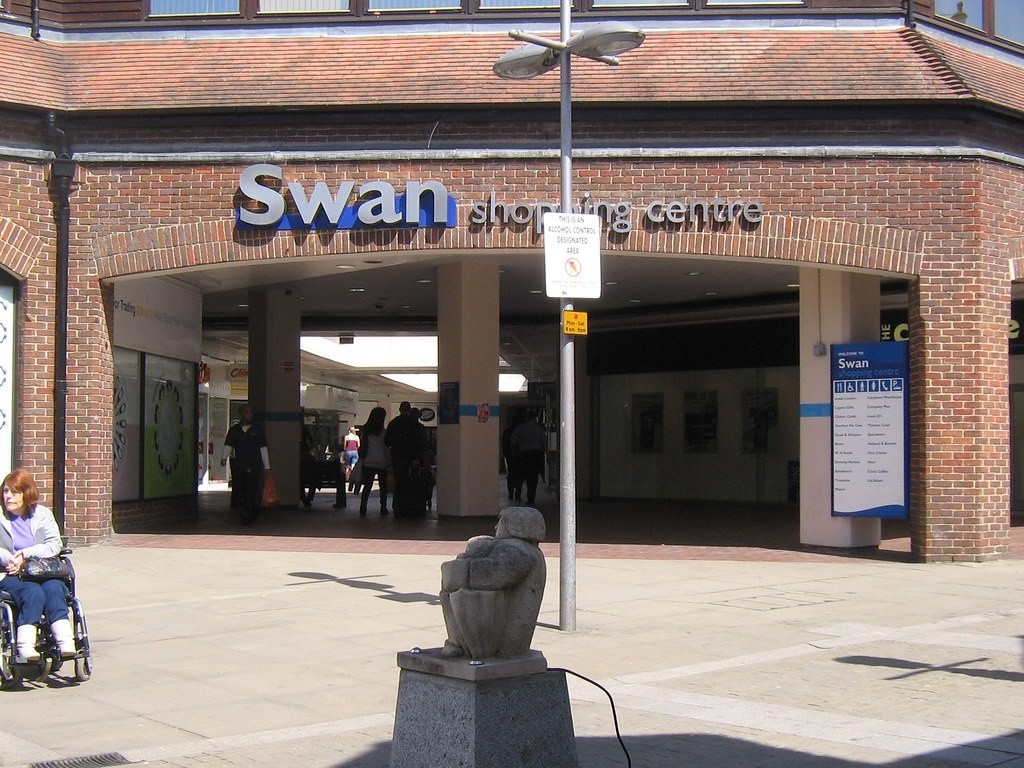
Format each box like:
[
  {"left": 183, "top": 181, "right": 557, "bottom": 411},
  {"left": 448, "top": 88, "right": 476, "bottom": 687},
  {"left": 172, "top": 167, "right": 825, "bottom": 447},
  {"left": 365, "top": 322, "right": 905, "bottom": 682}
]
[{"left": 300, "top": 458, "right": 347, "bottom": 508}]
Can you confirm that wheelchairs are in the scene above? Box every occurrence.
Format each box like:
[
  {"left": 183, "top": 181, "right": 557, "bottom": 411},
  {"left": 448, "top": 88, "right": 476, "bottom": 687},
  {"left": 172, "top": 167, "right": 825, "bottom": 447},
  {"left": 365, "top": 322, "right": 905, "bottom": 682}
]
[{"left": 0, "top": 548, "right": 93, "bottom": 683}]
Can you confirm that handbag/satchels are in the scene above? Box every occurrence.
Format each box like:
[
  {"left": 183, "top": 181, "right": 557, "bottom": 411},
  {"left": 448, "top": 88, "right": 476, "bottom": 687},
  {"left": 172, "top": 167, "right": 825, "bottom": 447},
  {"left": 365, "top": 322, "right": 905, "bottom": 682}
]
[
  {"left": 263, "top": 472, "right": 280, "bottom": 507},
  {"left": 19, "top": 547, "right": 70, "bottom": 581}
]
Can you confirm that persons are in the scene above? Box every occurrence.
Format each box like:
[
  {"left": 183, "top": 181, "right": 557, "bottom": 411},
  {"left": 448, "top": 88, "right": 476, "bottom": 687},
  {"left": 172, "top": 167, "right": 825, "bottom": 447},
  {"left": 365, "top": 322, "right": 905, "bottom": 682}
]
[
  {"left": 439, "top": 507, "right": 546, "bottom": 659},
  {"left": 0, "top": 469, "right": 76, "bottom": 661},
  {"left": 383, "top": 401, "right": 427, "bottom": 518},
  {"left": 510, "top": 410, "right": 549, "bottom": 506},
  {"left": 358, "top": 406, "right": 390, "bottom": 517},
  {"left": 300, "top": 441, "right": 323, "bottom": 508},
  {"left": 502, "top": 412, "right": 525, "bottom": 500},
  {"left": 221, "top": 404, "right": 271, "bottom": 526},
  {"left": 456, "top": 535, "right": 496, "bottom": 559},
  {"left": 344, "top": 427, "right": 360, "bottom": 482},
  {"left": 410, "top": 407, "right": 432, "bottom": 476}
]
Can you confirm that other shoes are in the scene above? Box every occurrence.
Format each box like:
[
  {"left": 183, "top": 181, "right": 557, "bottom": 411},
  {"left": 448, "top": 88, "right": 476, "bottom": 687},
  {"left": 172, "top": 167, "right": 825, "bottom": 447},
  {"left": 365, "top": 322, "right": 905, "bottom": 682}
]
[
  {"left": 381, "top": 506, "right": 388, "bottom": 514},
  {"left": 515, "top": 496, "right": 521, "bottom": 501},
  {"left": 508, "top": 494, "right": 513, "bottom": 499},
  {"left": 360, "top": 506, "right": 368, "bottom": 516},
  {"left": 526, "top": 500, "right": 535, "bottom": 507}
]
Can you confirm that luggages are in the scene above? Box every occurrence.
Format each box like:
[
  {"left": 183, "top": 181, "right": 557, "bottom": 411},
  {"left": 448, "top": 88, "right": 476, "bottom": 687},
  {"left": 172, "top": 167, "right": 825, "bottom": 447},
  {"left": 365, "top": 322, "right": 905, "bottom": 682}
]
[{"left": 392, "top": 463, "right": 426, "bottom": 518}]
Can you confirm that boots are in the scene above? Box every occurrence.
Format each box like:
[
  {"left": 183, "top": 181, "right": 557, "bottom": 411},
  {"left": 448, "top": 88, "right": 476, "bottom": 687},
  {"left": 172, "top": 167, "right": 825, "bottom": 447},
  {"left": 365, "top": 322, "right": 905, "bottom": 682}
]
[
  {"left": 17, "top": 624, "right": 40, "bottom": 661},
  {"left": 50, "top": 619, "right": 77, "bottom": 658}
]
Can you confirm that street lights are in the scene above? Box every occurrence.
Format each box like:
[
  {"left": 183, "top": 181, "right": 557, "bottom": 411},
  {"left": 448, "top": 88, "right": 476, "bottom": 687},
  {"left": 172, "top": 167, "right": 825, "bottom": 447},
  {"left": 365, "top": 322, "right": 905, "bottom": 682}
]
[{"left": 493, "top": 0, "right": 647, "bottom": 624}]
